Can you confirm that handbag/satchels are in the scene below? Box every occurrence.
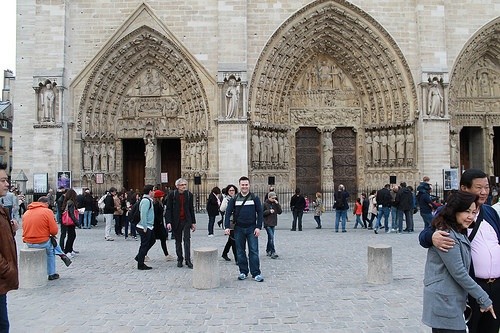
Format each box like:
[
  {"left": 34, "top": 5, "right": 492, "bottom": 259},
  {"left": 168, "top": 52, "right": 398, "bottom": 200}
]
[
  {"left": 50, "top": 236, "right": 57, "bottom": 247},
  {"left": 61, "top": 203, "right": 79, "bottom": 225},
  {"left": 229, "top": 219, "right": 236, "bottom": 240}
]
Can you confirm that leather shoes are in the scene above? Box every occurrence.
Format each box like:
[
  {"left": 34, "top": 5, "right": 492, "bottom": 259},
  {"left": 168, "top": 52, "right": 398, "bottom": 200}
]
[
  {"left": 186, "top": 261, "right": 193, "bottom": 267},
  {"left": 134, "top": 255, "right": 144, "bottom": 264},
  {"left": 178, "top": 261, "right": 183, "bottom": 267},
  {"left": 138, "top": 264, "right": 152, "bottom": 270},
  {"left": 221, "top": 255, "right": 231, "bottom": 261}
]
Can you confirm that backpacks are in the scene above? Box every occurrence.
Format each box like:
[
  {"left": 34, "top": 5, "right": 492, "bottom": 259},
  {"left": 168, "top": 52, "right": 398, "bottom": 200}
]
[{"left": 127, "top": 196, "right": 151, "bottom": 225}]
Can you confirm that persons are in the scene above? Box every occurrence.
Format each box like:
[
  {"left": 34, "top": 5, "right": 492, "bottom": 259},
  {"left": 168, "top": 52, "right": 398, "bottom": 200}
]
[
  {"left": 374, "top": 185, "right": 393, "bottom": 234},
  {"left": 54, "top": 183, "right": 172, "bottom": 242},
  {"left": 57, "top": 189, "right": 71, "bottom": 253},
  {"left": 334, "top": 184, "right": 350, "bottom": 233},
  {"left": 219, "top": 184, "right": 241, "bottom": 265},
  {"left": 0, "top": 188, "right": 15, "bottom": 219},
  {"left": 264, "top": 186, "right": 278, "bottom": 203},
  {"left": 389, "top": 184, "right": 400, "bottom": 233},
  {"left": 207, "top": 186, "right": 221, "bottom": 236},
  {"left": 217, "top": 188, "right": 227, "bottom": 227},
  {"left": 225, "top": 177, "right": 263, "bottom": 282},
  {"left": 395, "top": 183, "right": 414, "bottom": 233},
  {"left": 165, "top": 179, "right": 196, "bottom": 268},
  {"left": 9, "top": 186, "right": 19, "bottom": 217},
  {"left": 353, "top": 198, "right": 365, "bottom": 229},
  {"left": 361, "top": 192, "right": 373, "bottom": 229},
  {"left": 404, "top": 185, "right": 416, "bottom": 232},
  {"left": 17, "top": 190, "right": 27, "bottom": 217},
  {"left": 290, "top": 187, "right": 306, "bottom": 230},
  {"left": 418, "top": 175, "right": 432, "bottom": 197},
  {"left": 368, "top": 189, "right": 386, "bottom": 229},
  {"left": 133, "top": 185, "right": 156, "bottom": 270},
  {"left": 418, "top": 169, "right": 500, "bottom": 333},
  {"left": 263, "top": 192, "right": 282, "bottom": 259},
  {"left": 20, "top": 196, "right": 72, "bottom": 280},
  {"left": 419, "top": 183, "right": 434, "bottom": 230},
  {"left": 422, "top": 189, "right": 496, "bottom": 333},
  {"left": 313, "top": 191, "right": 323, "bottom": 229},
  {"left": 60, "top": 188, "right": 81, "bottom": 260},
  {"left": 144, "top": 189, "right": 177, "bottom": 262},
  {"left": 0, "top": 162, "right": 20, "bottom": 333}
]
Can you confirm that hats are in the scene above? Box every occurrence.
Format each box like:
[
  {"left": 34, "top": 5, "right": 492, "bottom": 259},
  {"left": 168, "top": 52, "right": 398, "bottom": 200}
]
[{"left": 153, "top": 190, "right": 165, "bottom": 197}]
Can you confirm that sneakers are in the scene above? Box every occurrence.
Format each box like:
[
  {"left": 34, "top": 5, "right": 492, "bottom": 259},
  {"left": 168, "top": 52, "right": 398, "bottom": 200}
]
[
  {"left": 71, "top": 251, "right": 79, "bottom": 256},
  {"left": 144, "top": 255, "right": 153, "bottom": 262},
  {"left": 66, "top": 252, "right": 74, "bottom": 258},
  {"left": 254, "top": 274, "right": 263, "bottom": 282},
  {"left": 270, "top": 253, "right": 279, "bottom": 259},
  {"left": 165, "top": 255, "right": 176, "bottom": 262},
  {"left": 49, "top": 274, "right": 59, "bottom": 280},
  {"left": 61, "top": 256, "right": 72, "bottom": 266},
  {"left": 267, "top": 251, "right": 272, "bottom": 256},
  {"left": 238, "top": 273, "right": 249, "bottom": 280}
]
